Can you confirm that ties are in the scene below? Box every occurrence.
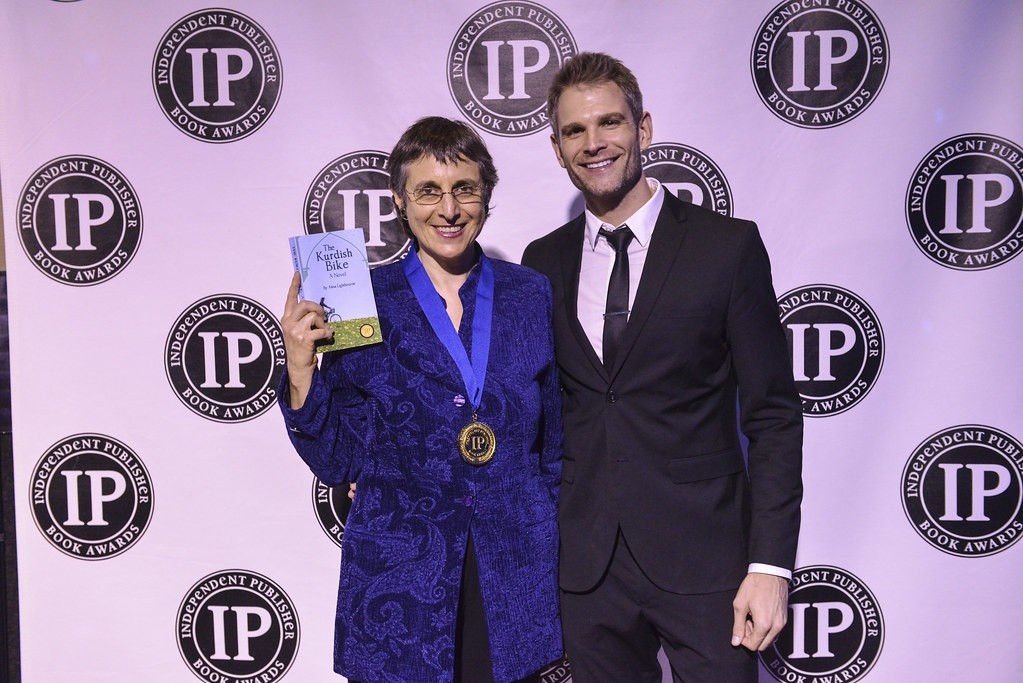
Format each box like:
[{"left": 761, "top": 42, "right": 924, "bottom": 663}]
[{"left": 598, "top": 227, "right": 634, "bottom": 376}]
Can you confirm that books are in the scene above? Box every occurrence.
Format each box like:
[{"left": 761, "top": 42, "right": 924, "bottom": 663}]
[{"left": 287, "top": 228, "right": 385, "bottom": 353}]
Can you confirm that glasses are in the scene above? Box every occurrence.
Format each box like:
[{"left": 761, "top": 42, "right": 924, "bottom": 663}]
[{"left": 404, "top": 184, "right": 486, "bottom": 203}]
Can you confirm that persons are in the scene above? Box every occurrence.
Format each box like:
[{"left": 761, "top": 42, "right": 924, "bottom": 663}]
[
  {"left": 519, "top": 52, "right": 803, "bottom": 683},
  {"left": 275, "top": 115, "right": 563, "bottom": 682}
]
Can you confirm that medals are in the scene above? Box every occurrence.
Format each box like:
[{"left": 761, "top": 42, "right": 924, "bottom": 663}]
[{"left": 458, "top": 421, "right": 497, "bottom": 466}]
